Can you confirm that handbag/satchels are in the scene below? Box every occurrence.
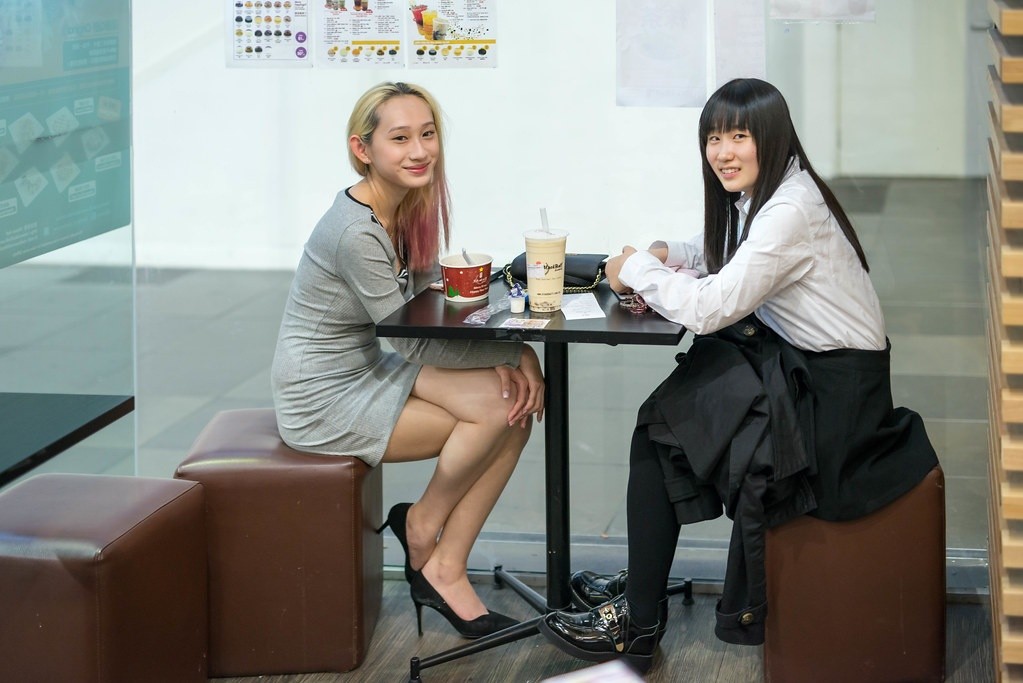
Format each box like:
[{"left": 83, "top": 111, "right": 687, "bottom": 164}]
[{"left": 502, "top": 250, "right": 609, "bottom": 295}]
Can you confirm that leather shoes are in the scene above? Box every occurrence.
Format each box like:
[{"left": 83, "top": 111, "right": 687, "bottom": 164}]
[
  {"left": 539, "top": 592, "right": 659, "bottom": 672},
  {"left": 568, "top": 570, "right": 669, "bottom": 645}
]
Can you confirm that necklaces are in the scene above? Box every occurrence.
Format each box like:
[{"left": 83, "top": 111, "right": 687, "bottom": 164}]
[{"left": 366, "top": 176, "right": 395, "bottom": 240}]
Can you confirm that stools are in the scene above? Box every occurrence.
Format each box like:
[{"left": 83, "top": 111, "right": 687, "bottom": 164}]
[
  {"left": 0, "top": 473, "right": 209, "bottom": 683},
  {"left": 763, "top": 462, "right": 948, "bottom": 683},
  {"left": 173, "top": 408, "right": 384, "bottom": 678}
]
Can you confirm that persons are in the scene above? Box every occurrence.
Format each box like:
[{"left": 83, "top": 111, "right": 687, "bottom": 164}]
[
  {"left": 537, "top": 77, "right": 942, "bottom": 677},
  {"left": 268, "top": 81, "right": 546, "bottom": 641}
]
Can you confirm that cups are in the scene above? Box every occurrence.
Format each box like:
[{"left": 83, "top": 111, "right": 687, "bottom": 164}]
[
  {"left": 326, "top": 0, "right": 345, "bottom": 10},
  {"left": 433, "top": 18, "right": 447, "bottom": 40},
  {"left": 354, "top": 0, "right": 368, "bottom": 11},
  {"left": 523, "top": 229, "right": 568, "bottom": 312},
  {"left": 410, "top": 5, "right": 428, "bottom": 35},
  {"left": 421, "top": 10, "right": 437, "bottom": 40}
]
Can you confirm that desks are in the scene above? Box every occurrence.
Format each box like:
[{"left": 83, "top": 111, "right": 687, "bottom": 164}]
[
  {"left": 1, "top": 391, "right": 135, "bottom": 490},
  {"left": 375, "top": 267, "right": 695, "bottom": 683}
]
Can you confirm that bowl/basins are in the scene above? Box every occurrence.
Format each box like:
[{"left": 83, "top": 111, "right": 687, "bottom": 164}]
[{"left": 439, "top": 253, "right": 493, "bottom": 302}]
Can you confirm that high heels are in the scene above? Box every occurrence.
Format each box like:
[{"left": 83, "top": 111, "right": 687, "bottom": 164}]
[
  {"left": 376, "top": 500, "right": 417, "bottom": 586},
  {"left": 410, "top": 569, "right": 519, "bottom": 639}
]
